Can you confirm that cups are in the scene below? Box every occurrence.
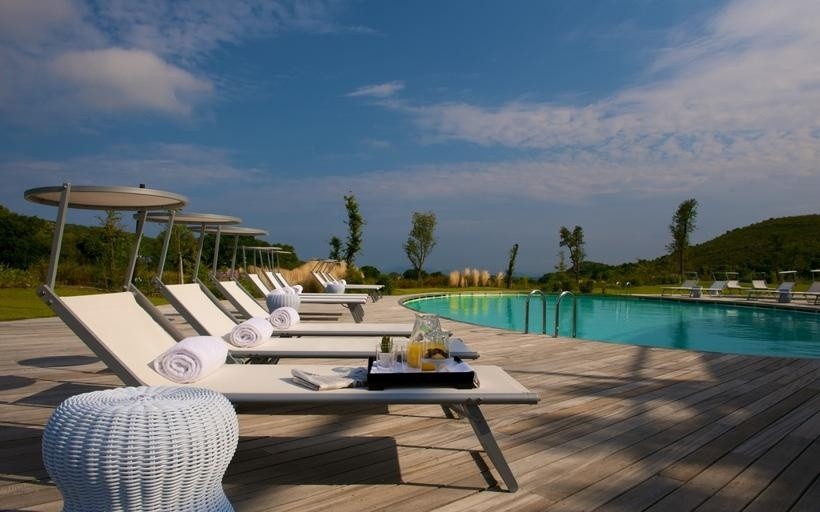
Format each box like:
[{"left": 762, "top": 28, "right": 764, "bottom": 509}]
[
  {"left": 422, "top": 333, "right": 449, "bottom": 360},
  {"left": 377, "top": 343, "right": 422, "bottom": 372}
]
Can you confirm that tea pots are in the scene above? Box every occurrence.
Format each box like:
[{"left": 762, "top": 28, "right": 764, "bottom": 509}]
[{"left": 407, "top": 313, "right": 445, "bottom": 370}]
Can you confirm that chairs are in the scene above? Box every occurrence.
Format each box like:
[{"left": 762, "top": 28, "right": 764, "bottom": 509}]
[
  {"left": 660, "top": 268, "right": 820, "bottom": 306},
  {"left": 21, "top": 183, "right": 542, "bottom": 493}
]
